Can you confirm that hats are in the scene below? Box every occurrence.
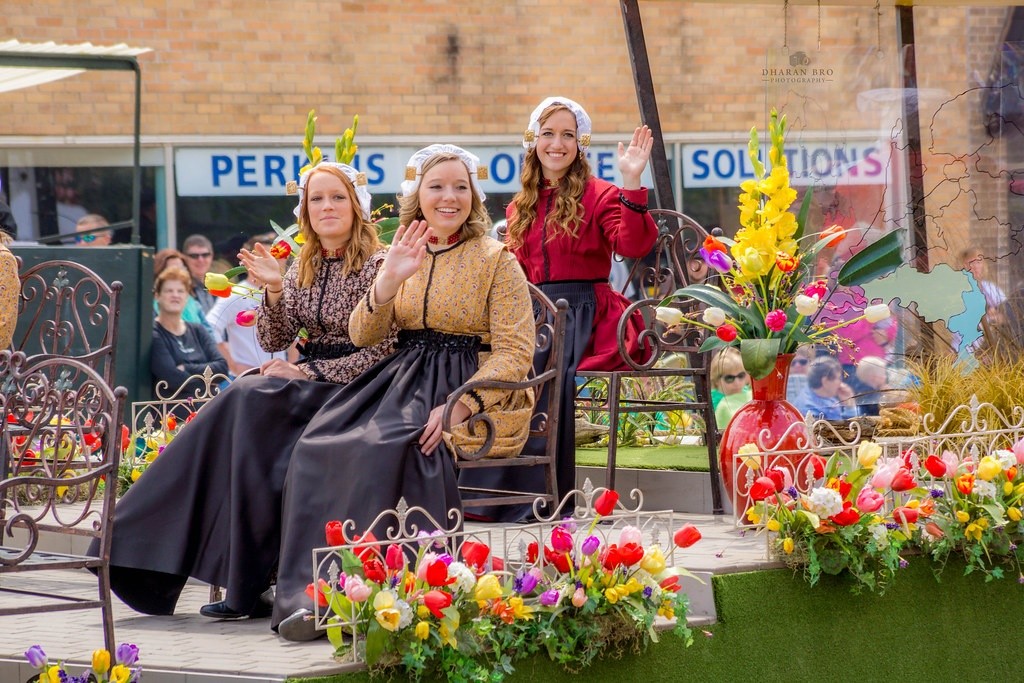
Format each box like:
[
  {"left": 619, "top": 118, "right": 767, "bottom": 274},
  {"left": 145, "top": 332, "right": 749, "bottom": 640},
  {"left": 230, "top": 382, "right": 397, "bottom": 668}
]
[{"left": 686, "top": 243, "right": 704, "bottom": 260}]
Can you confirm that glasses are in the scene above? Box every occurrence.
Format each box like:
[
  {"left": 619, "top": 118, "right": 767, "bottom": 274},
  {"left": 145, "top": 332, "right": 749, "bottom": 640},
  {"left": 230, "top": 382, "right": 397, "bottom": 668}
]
[
  {"left": 74, "top": 233, "right": 104, "bottom": 243},
  {"left": 723, "top": 371, "right": 746, "bottom": 383},
  {"left": 185, "top": 252, "right": 212, "bottom": 259},
  {"left": 790, "top": 358, "right": 808, "bottom": 366}
]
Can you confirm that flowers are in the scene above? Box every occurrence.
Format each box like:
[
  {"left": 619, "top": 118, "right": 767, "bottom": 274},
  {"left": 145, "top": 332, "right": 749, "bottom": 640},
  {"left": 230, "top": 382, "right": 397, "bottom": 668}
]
[
  {"left": 305, "top": 489, "right": 715, "bottom": 683},
  {"left": 24, "top": 642, "right": 143, "bottom": 683},
  {"left": 203, "top": 110, "right": 400, "bottom": 347},
  {"left": 4, "top": 397, "right": 196, "bottom": 506},
  {"left": 738, "top": 440, "right": 1024, "bottom": 597},
  {"left": 653, "top": 106, "right": 908, "bottom": 381}
]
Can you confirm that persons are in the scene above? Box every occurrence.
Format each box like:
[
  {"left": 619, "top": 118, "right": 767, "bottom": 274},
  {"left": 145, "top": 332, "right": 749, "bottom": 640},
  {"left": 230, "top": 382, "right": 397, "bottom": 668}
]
[
  {"left": 73, "top": 214, "right": 306, "bottom": 424},
  {"left": 271, "top": 142, "right": 536, "bottom": 642},
  {"left": 451, "top": 96, "right": 659, "bottom": 523},
  {"left": 603, "top": 221, "right": 1007, "bottom": 446},
  {"left": 80, "top": 161, "right": 402, "bottom": 619}
]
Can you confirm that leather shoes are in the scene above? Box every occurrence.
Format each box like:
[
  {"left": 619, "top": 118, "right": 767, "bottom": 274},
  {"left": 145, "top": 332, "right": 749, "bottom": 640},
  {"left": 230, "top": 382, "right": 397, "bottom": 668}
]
[{"left": 200, "top": 598, "right": 247, "bottom": 619}]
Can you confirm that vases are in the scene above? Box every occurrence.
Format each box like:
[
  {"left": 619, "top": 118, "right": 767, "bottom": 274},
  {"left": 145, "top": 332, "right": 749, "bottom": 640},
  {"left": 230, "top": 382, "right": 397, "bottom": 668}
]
[{"left": 719, "top": 353, "right": 819, "bottom": 529}]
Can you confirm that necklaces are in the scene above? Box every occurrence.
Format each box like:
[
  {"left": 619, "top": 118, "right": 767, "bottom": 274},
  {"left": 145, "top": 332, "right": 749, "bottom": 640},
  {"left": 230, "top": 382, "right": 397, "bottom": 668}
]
[
  {"left": 541, "top": 174, "right": 568, "bottom": 187},
  {"left": 322, "top": 243, "right": 349, "bottom": 257},
  {"left": 428, "top": 230, "right": 461, "bottom": 245}
]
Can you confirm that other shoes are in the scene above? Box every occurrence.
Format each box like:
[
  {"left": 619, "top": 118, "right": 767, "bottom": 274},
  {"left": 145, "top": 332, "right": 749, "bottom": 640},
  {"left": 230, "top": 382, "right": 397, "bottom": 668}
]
[{"left": 278, "top": 608, "right": 327, "bottom": 642}]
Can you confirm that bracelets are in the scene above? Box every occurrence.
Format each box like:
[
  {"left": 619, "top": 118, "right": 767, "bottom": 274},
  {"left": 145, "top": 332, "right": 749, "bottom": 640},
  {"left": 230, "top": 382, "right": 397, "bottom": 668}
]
[
  {"left": 619, "top": 193, "right": 648, "bottom": 213},
  {"left": 267, "top": 288, "right": 282, "bottom": 293}
]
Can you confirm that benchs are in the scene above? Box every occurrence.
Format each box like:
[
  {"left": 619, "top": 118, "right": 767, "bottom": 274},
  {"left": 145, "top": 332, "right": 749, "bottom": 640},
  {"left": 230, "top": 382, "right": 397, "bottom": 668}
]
[
  {"left": 234, "top": 278, "right": 569, "bottom": 525},
  {"left": 0, "top": 253, "right": 128, "bottom": 674},
  {"left": 573, "top": 209, "right": 724, "bottom": 515}
]
